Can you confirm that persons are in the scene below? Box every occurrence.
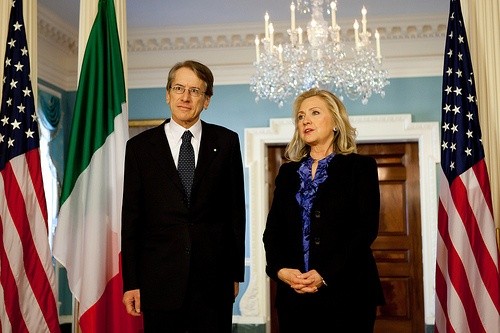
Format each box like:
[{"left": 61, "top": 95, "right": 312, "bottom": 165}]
[
  {"left": 262, "top": 87, "right": 384, "bottom": 333},
  {"left": 121, "top": 60, "right": 246, "bottom": 333}
]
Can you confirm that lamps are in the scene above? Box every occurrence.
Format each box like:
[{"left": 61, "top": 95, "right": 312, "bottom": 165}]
[{"left": 249, "top": 0, "right": 390, "bottom": 109}]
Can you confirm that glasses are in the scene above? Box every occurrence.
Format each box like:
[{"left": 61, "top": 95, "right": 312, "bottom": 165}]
[{"left": 170, "top": 86, "right": 206, "bottom": 97}]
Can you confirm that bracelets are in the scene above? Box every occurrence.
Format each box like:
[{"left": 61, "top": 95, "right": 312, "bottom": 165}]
[{"left": 322, "top": 278, "right": 327, "bottom": 286}]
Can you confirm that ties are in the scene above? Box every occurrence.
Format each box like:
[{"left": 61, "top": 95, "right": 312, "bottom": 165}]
[{"left": 178, "top": 130, "right": 195, "bottom": 258}]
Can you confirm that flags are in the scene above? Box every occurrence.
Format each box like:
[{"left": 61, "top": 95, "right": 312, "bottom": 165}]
[
  {"left": 434, "top": 0, "right": 500, "bottom": 333},
  {"left": 52, "top": 0, "right": 130, "bottom": 333},
  {"left": 0, "top": 0, "right": 59, "bottom": 333}
]
